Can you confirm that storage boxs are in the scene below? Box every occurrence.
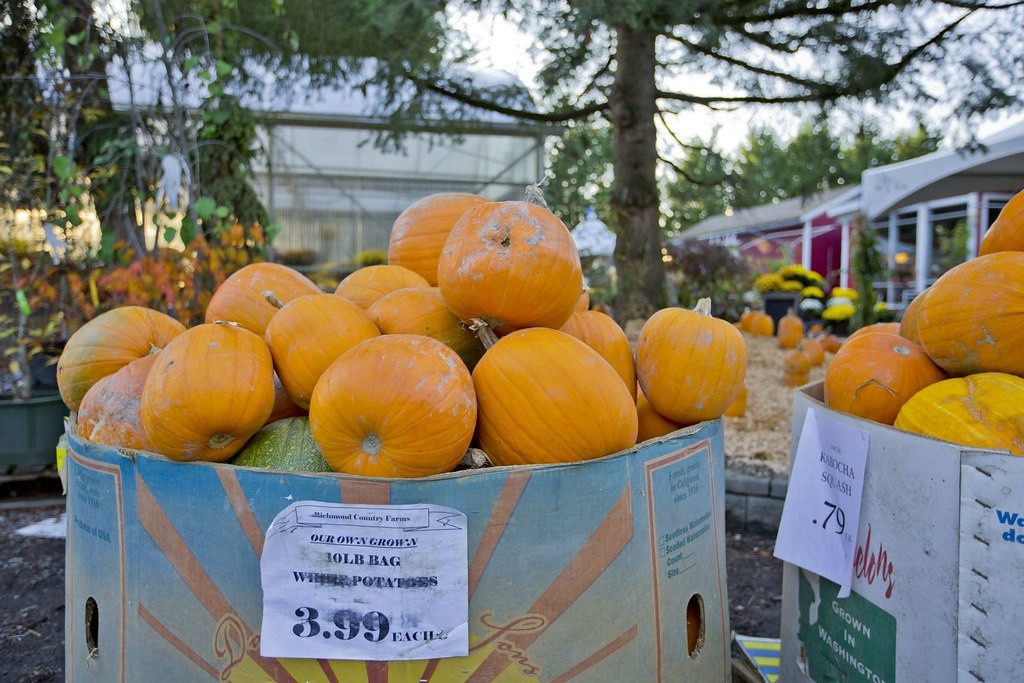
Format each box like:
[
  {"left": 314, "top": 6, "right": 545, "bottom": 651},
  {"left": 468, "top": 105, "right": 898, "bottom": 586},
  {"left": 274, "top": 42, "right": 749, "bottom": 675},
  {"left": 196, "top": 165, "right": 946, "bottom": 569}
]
[
  {"left": 64, "top": 405, "right": 732, "bottom": 683},
  {"left": 780, "top": 376, "right": 1024, "bottom": 682}
]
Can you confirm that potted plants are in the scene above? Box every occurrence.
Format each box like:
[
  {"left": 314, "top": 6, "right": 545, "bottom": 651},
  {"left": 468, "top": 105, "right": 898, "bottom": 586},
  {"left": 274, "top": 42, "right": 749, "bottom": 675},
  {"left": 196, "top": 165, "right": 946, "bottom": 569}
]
[{"left": 0, "top": 245, "right": 104, "bottom": 474}]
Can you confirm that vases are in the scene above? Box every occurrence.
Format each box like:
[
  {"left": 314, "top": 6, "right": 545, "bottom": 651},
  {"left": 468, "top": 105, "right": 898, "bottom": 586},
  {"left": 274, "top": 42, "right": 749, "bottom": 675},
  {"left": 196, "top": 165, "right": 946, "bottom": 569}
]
[
  {"left": 803, "top": 311, "right": 818, "bottom": 324},
  {"left": 832, "top": 319, "right": 849, "bottom": 335},
  {"left": 764, "top": 296, "right": 799, "bottom": 336}
]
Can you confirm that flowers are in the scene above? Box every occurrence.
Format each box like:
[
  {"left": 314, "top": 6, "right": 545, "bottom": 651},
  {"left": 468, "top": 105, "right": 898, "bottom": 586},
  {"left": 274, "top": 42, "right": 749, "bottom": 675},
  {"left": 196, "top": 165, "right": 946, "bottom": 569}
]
[{"left": 757, "top": 264, "right": 860, "bottom": 321}]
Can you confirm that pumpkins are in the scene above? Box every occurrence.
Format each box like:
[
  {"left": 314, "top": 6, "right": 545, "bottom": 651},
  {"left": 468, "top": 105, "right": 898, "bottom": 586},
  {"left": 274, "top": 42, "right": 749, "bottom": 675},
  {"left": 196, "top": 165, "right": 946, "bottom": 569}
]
[
  {"left": 730, "top": 185, "right": 1024, "bottom": 459},
  {"left": 56, "top": 191, "right": 749, "bottom": 478}
]
